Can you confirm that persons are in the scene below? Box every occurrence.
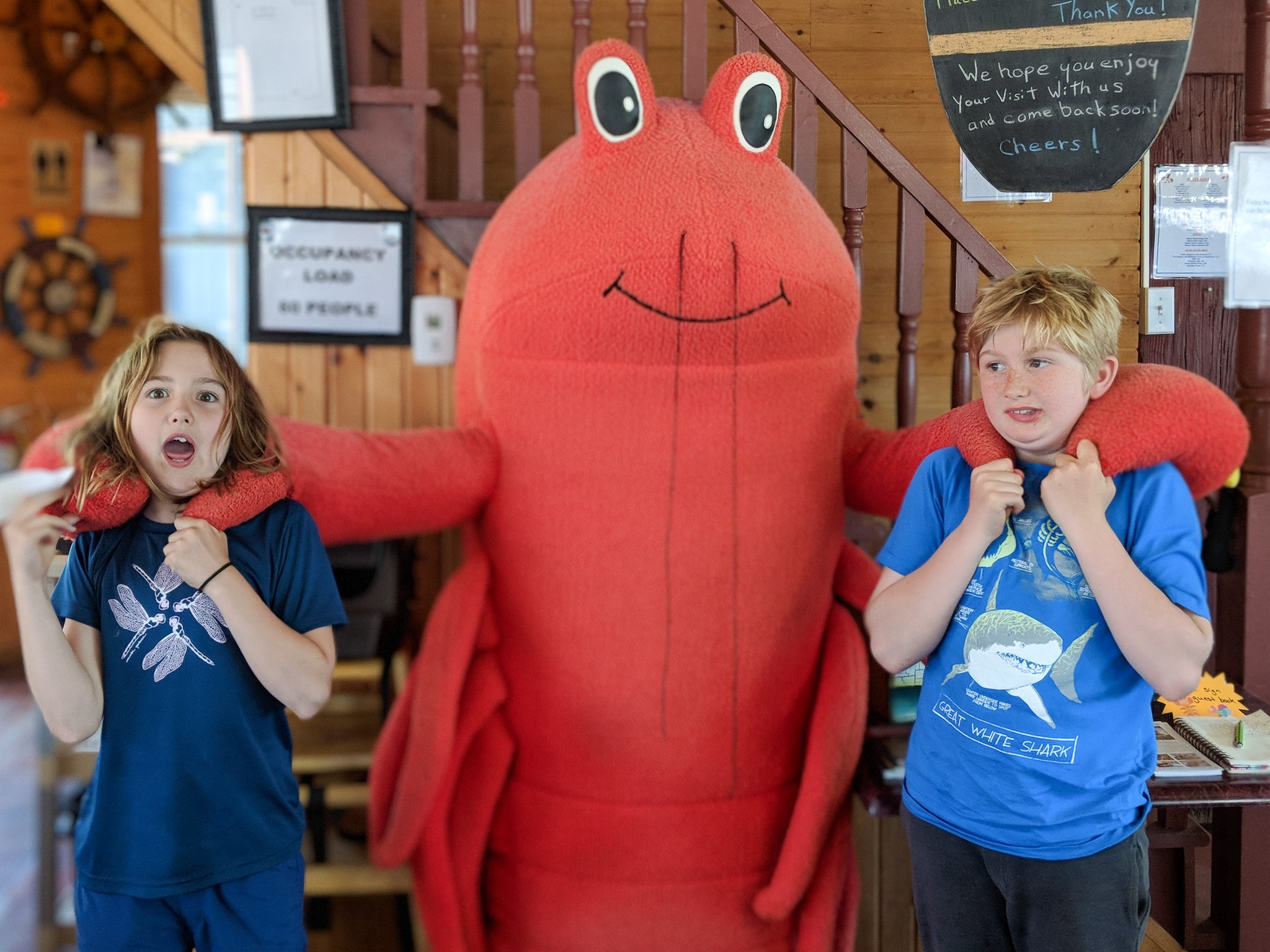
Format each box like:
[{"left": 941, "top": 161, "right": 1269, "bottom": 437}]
[
  {"left": 3, "top": 324, "right": 351, "bottom": 952},
  {"left": 864, "top": 269, "right": 1214, "bottom": 952}
]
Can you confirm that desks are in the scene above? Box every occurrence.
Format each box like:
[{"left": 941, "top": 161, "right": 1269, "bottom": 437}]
[{"left": 852, "top": 679, "right": 1270, "bottom": 951}]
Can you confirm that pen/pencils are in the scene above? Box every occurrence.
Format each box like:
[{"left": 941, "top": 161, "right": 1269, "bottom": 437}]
[{"left": 1235, "top": 720, "right": 1243, "bottom": 747}]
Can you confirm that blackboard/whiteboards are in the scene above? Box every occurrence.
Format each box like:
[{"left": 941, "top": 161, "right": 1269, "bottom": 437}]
[{"left": 923, "top": 0, "right": 1198, "bottom": 193}]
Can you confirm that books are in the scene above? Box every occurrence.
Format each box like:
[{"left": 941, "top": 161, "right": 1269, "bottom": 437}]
[{"left": 1154, "top": 710, "right": 1270, "bottom": 776}]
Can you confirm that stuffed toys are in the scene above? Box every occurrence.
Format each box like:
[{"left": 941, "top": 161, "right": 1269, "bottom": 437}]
[{"left": 21, "top": 37, "right": 1248, "bottom": 952}]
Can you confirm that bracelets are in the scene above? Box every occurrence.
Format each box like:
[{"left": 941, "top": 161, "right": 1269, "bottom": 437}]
[{"left": 198, "top": 561, "right": 234, "bottom": 591}]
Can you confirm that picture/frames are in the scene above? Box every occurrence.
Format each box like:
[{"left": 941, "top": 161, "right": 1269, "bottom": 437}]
[
  {"left": 245, "top": 205, "right": 415, "bottom": 346},
  {"left": 197, "top": 0, "right": 355, "bottom": 134}
]
